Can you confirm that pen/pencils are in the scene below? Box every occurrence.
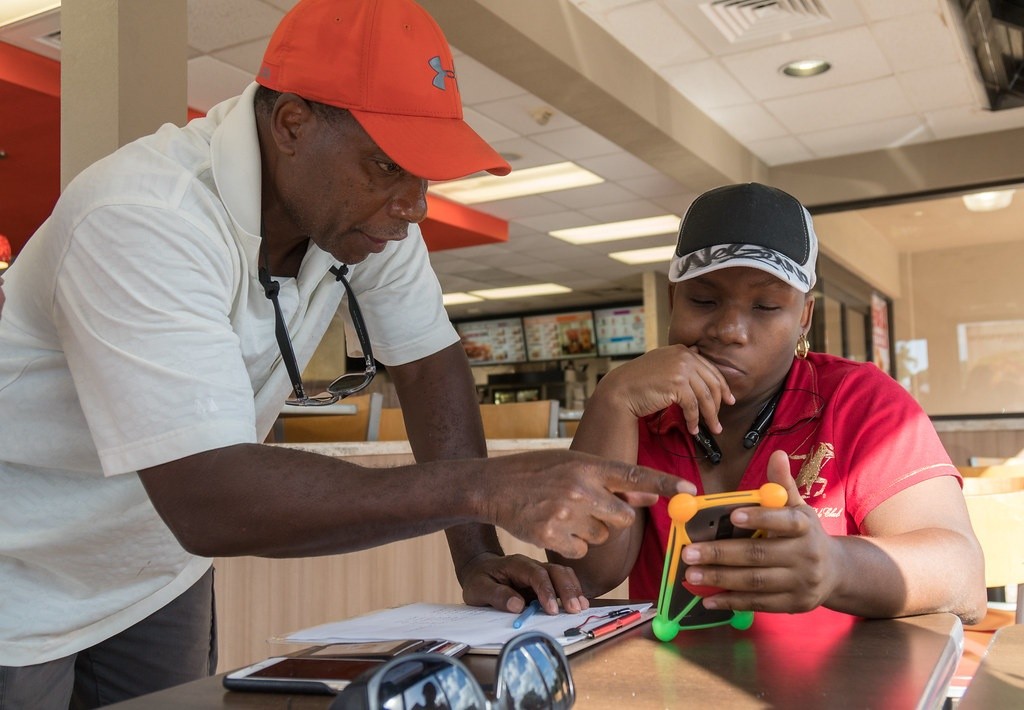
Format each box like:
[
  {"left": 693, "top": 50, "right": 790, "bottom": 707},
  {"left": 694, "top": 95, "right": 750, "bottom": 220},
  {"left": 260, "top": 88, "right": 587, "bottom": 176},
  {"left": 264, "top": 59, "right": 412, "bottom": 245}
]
[{"left": 512, "top": 599, "right": 541, "bottom": 629}]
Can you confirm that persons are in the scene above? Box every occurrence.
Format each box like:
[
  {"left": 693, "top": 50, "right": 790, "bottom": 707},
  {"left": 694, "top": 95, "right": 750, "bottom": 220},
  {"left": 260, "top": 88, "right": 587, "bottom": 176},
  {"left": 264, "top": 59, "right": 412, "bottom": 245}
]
[
  {"left": 545, "top": 181, "right": 987, "bottom": 624},
  {"left": 0, "top": 0, "right": 698, "bottom": 710}
]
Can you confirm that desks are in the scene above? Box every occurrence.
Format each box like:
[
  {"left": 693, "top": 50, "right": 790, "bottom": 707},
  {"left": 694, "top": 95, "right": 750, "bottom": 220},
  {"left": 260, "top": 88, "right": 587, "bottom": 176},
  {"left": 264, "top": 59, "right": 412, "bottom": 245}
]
[
  {"left": 92, "top": 598, "right": 966, "bottom": 710},
  {"left": 950, "top": 619, "right": 1024, "bottom": 710},
  {"left": 278, "top": 404, "right": 357, "bottom": 418}
]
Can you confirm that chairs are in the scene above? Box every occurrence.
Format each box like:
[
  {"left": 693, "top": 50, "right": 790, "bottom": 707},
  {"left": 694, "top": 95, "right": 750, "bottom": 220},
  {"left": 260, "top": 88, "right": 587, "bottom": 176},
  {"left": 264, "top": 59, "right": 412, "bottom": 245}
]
[
  {"left": 273, "top": 391, "right": 562, "bottom": 442},
  {"left": 956, "top": 450, "right": 1024, "bottom": 624}
]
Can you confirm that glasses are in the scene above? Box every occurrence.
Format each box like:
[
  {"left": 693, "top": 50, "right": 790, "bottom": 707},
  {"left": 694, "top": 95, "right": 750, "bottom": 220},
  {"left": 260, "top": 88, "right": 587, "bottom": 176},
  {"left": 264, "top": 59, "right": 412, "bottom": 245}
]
[
  {"left": 328, "top": 631, "right": 576, "bottom": 710},
  {"left": 257, "top": 259, "right": 386, "bottom": 407}
]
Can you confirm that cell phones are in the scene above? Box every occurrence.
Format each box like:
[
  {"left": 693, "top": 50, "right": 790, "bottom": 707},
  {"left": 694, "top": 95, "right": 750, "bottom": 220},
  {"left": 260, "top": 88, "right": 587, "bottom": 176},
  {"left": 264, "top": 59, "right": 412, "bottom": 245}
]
[
  {"left": 652, "top": 482, "right": 789, "bottom": 641},
  {"left": 222, "top": 655, "right": 388, "bottom": 694}
]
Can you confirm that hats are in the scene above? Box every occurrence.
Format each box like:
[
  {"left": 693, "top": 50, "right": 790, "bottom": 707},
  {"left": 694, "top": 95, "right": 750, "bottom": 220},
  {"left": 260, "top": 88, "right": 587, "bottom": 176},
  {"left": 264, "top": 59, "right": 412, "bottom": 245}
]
[
  {"left": 667, "top": 181, "right": 819, "bottom": 293},
  {"left": 256, "top": 0, "right": 511, "bottom": 183}
]
